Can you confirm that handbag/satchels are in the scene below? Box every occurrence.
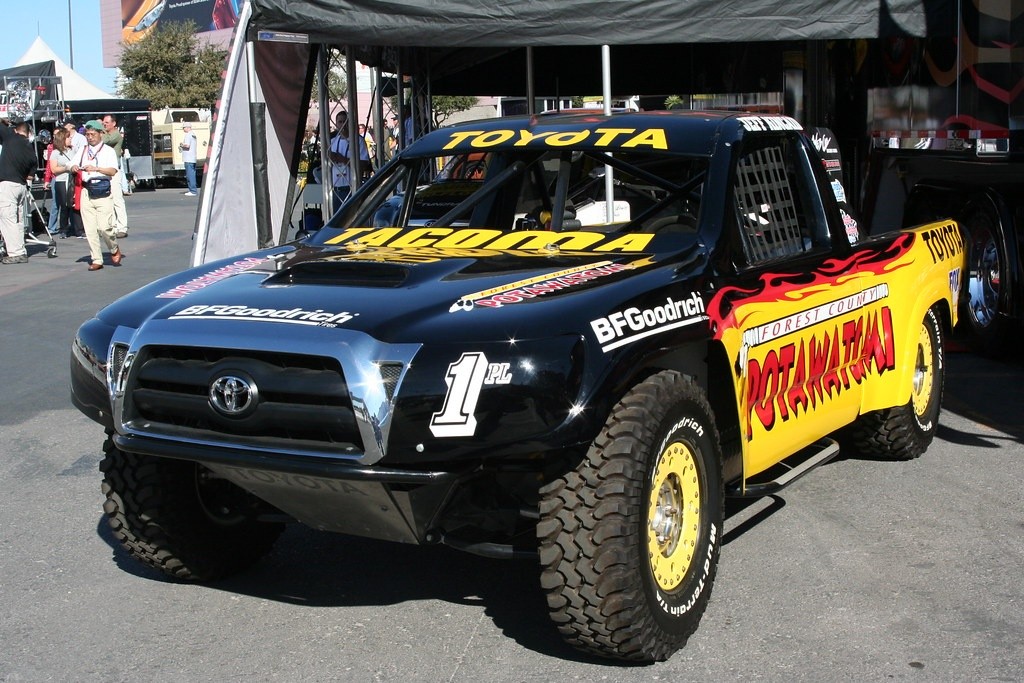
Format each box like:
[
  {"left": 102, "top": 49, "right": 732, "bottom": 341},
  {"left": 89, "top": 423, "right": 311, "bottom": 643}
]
[{"left": 78, "top": 176, "right": 111, "bottom": 199}]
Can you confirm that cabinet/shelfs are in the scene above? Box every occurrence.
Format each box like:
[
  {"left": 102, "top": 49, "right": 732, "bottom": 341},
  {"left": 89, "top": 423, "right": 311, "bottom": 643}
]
[{"left": 303, "top": 184, "right": 323, "bottom": 234}]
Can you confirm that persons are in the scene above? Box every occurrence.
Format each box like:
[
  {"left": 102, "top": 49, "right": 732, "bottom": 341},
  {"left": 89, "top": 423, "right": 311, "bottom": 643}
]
[
  {"left": 301, "top": 103, "right": 412, "bottom": 215},
  {"left": 0, "top": 115, "right": 129, "bottom": 271},
  {"left": 179, "top": 122, "right": 197, "bottom": 196}
]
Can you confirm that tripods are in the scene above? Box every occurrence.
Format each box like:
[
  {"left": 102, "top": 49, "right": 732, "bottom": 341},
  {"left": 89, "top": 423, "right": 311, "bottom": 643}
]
[{"left": 17, "top": 180, "right": 57, "bottom": 259}]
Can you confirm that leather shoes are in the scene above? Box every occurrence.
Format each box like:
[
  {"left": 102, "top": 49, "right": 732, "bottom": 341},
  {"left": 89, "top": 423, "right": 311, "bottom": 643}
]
[
  {"left": 110, "top": 247, "right": 121, "bottom": 265},
  {"left": 88, "top": 263, "right": 103, "bottom": 271}
]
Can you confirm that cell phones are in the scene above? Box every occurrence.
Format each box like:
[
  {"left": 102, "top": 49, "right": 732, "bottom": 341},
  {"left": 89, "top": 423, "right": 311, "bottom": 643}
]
[{"left": 79, "top": 167, "right": 86, "bottom": 171}]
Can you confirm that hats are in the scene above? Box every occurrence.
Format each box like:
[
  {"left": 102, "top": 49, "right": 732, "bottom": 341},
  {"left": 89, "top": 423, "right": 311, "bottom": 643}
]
[
  {"left": 85, "top": 120, "right": 105, "bottom": 134},
  {"left": 180, "top": 122, "right": 192, "bottom": 128},
  {"left": 391, "top": 114, "right": 398, "bottom": 120}
]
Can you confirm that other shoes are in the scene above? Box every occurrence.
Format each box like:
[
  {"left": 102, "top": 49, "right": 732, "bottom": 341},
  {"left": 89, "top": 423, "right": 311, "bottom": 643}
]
[
  {"left": 47, "top": 230, "right": 60, "bottom": 235},
  {"left": 59, "top": 232, "right": 68, "bottom": 239},
  {"left": 116, "top": 232, "right": 128, "bottom": 238},
  {"left": 2, "top": 255, "right": 28, "bottom": 264},
  {"left": 76, "top": 233, "right": 87, "bottom": 239},
  {"left": 185, "top": 192, "right": 197, "bottom": 196}
]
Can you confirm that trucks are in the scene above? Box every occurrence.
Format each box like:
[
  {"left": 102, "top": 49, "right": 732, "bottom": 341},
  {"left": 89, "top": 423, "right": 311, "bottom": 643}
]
[{"left": 56, "top": 99, "right": 212, "bottom": 190}]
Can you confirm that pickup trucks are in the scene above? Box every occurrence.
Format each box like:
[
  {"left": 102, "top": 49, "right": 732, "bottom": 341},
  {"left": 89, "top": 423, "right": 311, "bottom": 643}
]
[
  {"left": 856, "top": 129, "right": 1023, "bottom": 363},
  {"left": 71, "top": 108, "right": 964, "bottom": 664}
]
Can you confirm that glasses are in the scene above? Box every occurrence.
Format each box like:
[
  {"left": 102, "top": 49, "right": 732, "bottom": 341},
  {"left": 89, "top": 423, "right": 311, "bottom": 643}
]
[{"left": 86, "top": 133, "right": 97, "bottom": 136}]
